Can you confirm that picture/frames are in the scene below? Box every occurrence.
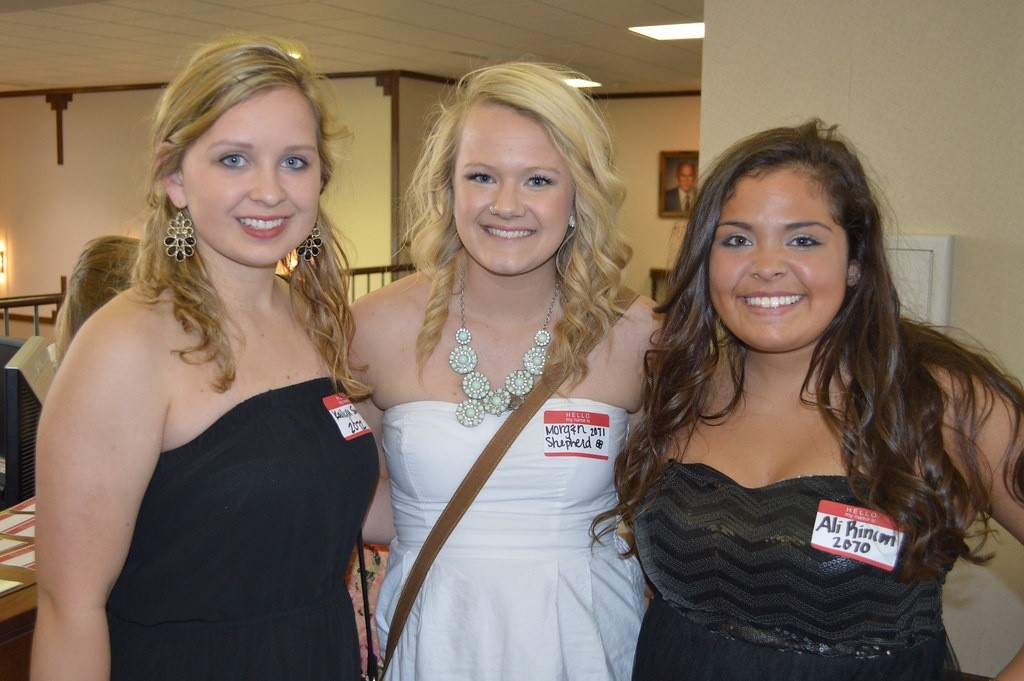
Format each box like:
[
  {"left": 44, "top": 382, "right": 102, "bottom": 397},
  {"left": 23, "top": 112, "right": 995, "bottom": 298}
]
[{"left": 659, "top": 151, "right": 699, "bottom": 218}]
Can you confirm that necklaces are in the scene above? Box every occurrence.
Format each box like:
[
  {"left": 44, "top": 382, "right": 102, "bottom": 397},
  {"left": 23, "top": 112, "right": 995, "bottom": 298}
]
[{"left": 449, "top": 267, "right": 559, "bottom": 426}]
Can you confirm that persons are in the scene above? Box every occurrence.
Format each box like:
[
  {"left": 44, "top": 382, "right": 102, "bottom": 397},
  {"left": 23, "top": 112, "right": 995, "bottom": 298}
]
[
  {"left": 48, "top": 237, "right": 141, "bottom": 367},
  {"left": 332, "top": 60, "right": 670, "bottom": 681},
  {"left": 667, "top": 161, "right": 698, "bottom": 211},
  {"left": 31, "top": 34, "right": 395, "bottom": 681},
  {"left": 612, "top": 118, "right": 1024, "bottom": 680}
]
